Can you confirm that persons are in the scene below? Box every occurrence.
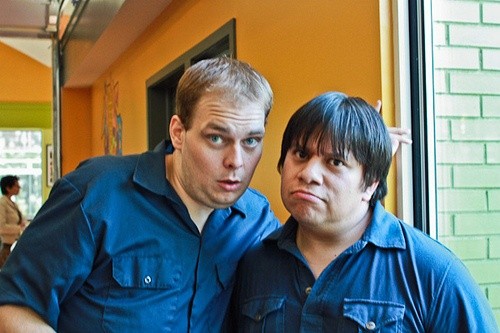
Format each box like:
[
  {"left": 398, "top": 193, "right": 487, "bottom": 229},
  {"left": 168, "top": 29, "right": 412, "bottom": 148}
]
[
  {"left": 0, "top": 55, "right": 412, "bottom": 333},
  {"left": 231, "top": 92, "right": 499, "bottom": 333},
  {"left": 0, "top": 176, "right": 29, "bottom": 266}
]
